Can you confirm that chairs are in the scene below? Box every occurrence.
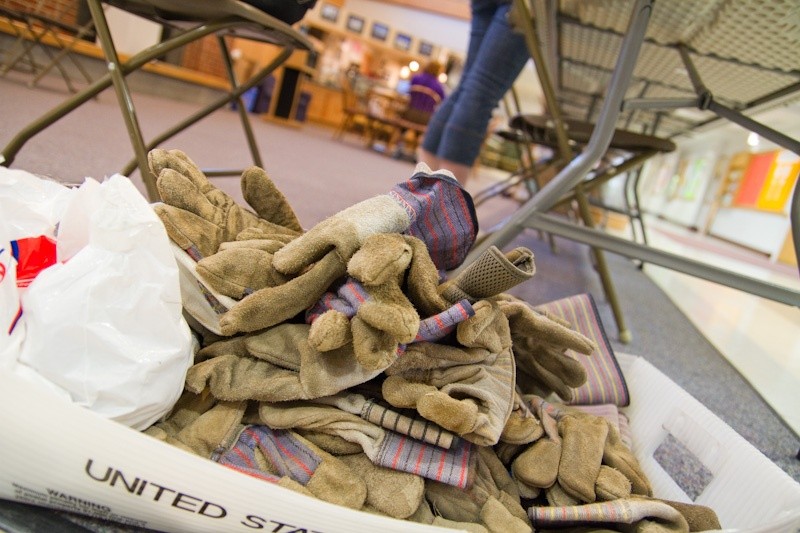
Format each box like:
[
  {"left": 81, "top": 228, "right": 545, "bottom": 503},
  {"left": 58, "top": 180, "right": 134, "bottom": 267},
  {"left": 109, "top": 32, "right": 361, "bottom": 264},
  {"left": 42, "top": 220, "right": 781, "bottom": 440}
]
[{"left": 0, "top": 0, "right": 678, "bottom": 346}]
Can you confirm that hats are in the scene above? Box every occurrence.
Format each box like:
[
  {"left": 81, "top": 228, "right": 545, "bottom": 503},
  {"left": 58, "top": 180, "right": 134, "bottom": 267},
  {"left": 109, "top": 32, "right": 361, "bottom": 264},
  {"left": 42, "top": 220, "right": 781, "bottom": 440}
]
[{"left": 423, "top": 61, "right": 440, "bottom": 76}]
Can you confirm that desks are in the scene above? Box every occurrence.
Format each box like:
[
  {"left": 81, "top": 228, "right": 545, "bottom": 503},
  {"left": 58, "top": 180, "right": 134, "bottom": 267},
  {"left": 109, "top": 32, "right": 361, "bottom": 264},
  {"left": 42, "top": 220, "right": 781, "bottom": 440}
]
[
  {"left": 364, "top": 87, "right": 471, "bottom": 154},
  {"left": 450, "top": 0, "right": 800, "bottom": 423}
]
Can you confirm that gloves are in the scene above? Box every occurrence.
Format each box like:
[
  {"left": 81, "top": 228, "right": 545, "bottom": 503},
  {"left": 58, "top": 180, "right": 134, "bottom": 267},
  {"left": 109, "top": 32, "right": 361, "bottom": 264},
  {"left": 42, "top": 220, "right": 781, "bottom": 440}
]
[{"left": 146, "top": 141, "right": 723, "bottom": 533}]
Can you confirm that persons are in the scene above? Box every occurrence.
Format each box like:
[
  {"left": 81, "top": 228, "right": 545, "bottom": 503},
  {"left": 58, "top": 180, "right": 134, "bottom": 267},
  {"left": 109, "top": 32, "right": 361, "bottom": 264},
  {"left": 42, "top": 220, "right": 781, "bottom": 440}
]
[
  {"left": 394, "top": 61, "right": 447, "bottom": 164},
  {"left": 418, "top": 0, "right": 531, "bottom": 190}
]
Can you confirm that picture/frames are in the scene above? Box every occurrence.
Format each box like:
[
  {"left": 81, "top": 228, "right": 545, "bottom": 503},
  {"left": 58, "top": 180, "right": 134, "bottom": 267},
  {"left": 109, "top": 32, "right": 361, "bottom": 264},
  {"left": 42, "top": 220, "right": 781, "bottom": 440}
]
[
  {"left": 420, "top": 42, "right": 433, "bottom": 56},
  {"left": 395, "top": 33, "right": 411, "bottom": 51},
  {"left": 322, "top": 4, "right": 340, "bottom": 22},
  {"left": 370, "top": 23, "right": 388, "bottom": 39},
  {"left": 346, "top": 16, "right": 364, "bottom": 34}
]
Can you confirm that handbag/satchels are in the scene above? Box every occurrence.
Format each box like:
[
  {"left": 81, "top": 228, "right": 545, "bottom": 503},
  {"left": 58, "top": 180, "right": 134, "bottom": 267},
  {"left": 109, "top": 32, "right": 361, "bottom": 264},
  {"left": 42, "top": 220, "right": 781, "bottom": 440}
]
[{"left": 367, "top": 96, "right": 387, "bottom": 118}]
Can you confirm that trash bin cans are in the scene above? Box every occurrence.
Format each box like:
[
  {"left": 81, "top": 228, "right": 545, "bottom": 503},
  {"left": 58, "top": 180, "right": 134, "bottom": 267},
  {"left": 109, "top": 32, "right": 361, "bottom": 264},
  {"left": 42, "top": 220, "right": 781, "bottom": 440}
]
[{"left": 254, "top": 62, "right": 315, "bottom": 131}]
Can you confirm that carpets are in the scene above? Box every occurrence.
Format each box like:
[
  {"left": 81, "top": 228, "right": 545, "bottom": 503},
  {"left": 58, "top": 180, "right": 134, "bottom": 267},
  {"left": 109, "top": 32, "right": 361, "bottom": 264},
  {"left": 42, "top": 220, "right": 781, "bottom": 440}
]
[{"left": 441, "top": 211, "right": 800, "bottom": 484}]
